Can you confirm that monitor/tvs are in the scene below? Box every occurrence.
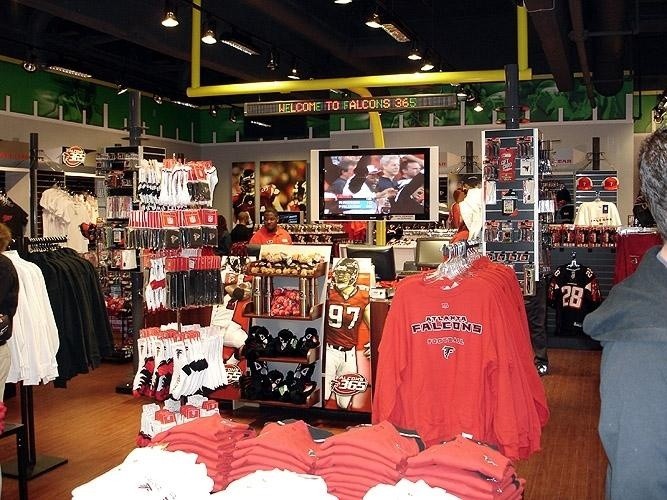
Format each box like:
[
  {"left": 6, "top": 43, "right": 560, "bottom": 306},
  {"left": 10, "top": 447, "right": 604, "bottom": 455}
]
[
  {"left": 310, "top": 146, "right": 440, "bottom": 222},
  {"left": 277, "top": 211, "right": 304, "bottom": 224}
]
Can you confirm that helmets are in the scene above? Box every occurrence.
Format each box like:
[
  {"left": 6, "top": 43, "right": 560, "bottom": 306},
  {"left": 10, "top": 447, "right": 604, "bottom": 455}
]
[
  {"left": 292, "top": 180, "right": 306, "bottom": 205},
  {"left": 332, "top": 258, "right": 360, "bottom": 289},
  {"left": 238, "top": 169, "right": 259, "bottom": 195}
]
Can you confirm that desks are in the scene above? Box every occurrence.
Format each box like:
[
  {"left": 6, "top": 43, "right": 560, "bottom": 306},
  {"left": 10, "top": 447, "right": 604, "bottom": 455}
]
[
  {"left": 369, "top": 298, "right": 394, "bottom": 409},
  {"left": 614, "top": 227, "right": 663, "bottom": 284}
]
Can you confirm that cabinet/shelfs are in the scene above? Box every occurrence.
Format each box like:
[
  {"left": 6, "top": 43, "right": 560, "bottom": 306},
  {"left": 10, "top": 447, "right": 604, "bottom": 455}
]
[
  {"left": 105, "top": 145, "right": 165, "bottom": 197},
  {"left": 32, "top": 168, "right": 67, "bottom": 237},
  {"left": 83, "top": 207, "right": 223, "bottom": 395},
  {"left": 482, "top": 128, "right": 539, "bottom": 281},
  {"left": 236, "top": 242, "right": 334, "bottom": 411},
  {"left": 0, "top": 166, "right": 32, "bottom": 251},
  {"left": 539, "top": 168, "right": 619, "bottom": 351}
]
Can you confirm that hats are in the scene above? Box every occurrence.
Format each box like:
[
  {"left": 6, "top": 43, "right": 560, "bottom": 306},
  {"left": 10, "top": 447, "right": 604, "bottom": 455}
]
[
  {"left": 574, "top": 176, "right": 592, "bottom": 191},
  {"left": 601, "top": 176, "right": 620, "bottom": 192},
  {"left": 362, "top": 164, "right": 384, "bottom": 176}
]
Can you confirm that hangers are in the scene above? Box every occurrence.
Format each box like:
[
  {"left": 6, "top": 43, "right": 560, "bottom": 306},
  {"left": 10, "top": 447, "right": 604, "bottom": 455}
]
[
  {"left": 424, "top": 235, "right": 481, "bottom": 287},
  {"left": 593, "top": 191, "right": 602, "bottom": 202},
  {"left": 27, "top": 235, "right": 71, "bottom": 253},
  {"left": 4, "top": 235, "right": 17, "bottom": 251},
  {"left": 565, "top": 252, "right": 580, "bottom": 272}
]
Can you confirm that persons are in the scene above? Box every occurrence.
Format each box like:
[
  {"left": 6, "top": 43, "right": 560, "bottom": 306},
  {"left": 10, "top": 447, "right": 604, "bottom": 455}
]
[
  {"left": 249, "top": 208, "right": 293, "bottom": 245},
  {"left": 230, "top": 211, "right": 253, "bottom": 242},
  {"left": 325, "top": 258, "right": 371, "bottom": 409},
  {"left": 582, "top": 129, "right": 666, "bottom": 500},
  {"left": 323, "top": 154, "right": 425, "bottom": 214},
  {"left": 0, "top": 223, "right": 19, "bottom": 403},
  {"left": 209, "top": 256, "right": 256, "bottom": 388},
  {"left": 233, "top": 169, "right": 283, "bottom": 223},
  {"left": 214, "top": 215, "right": 232, "bottom": 255}
]
